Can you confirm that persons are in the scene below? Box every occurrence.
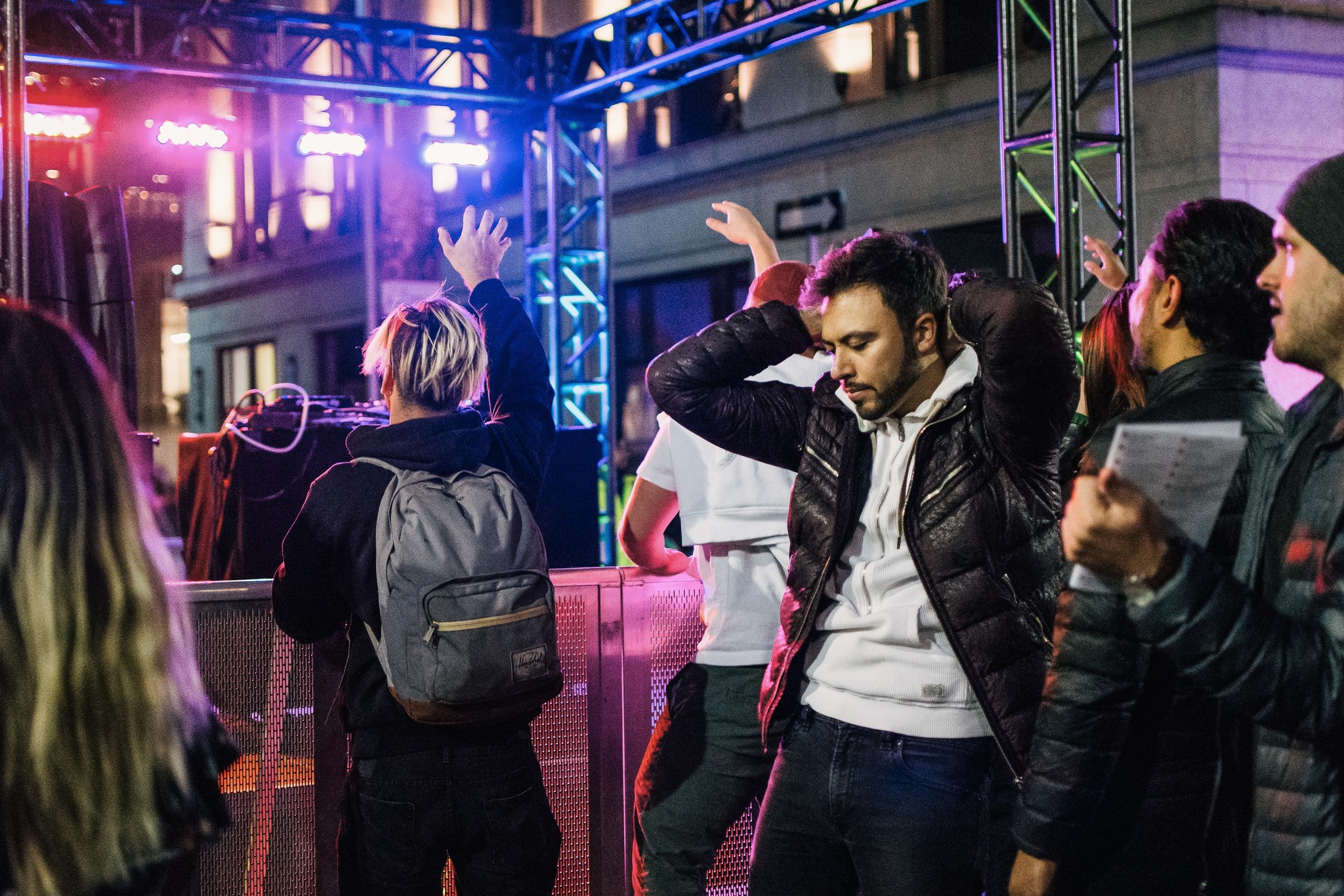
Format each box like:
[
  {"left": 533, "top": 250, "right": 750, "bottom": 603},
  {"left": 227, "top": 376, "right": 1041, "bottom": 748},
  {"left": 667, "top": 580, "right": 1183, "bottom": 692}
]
[
  {"left": 618, "top": 197, "right": 838, "bottom": 895},
  {"left": 1059, "top": 155, "right": 1344, "bottom": 896},
  {"left": 644, "top": 226, "right": 1080, "bottom": 895},
  {"left": 270, "top": 205, "right": 562, "bottom": 895},
  {"left": 0, "top": 296, "right": 239, "bottom": 896},
  {"left": 942, "top": 193, "right": 1288, "bottom": 896}
]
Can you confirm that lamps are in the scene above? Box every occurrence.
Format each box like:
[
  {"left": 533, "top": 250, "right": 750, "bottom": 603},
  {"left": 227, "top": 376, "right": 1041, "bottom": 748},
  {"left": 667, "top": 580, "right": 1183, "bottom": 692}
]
[{"left": 833, "top": 72, "right": 849, "bottom": 99}]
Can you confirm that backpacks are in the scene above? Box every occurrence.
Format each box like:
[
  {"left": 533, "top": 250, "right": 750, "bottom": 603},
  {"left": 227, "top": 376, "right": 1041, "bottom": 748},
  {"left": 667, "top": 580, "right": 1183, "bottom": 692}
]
[{"left": 351, "top": 457, "right": 564, "bottom": 726}]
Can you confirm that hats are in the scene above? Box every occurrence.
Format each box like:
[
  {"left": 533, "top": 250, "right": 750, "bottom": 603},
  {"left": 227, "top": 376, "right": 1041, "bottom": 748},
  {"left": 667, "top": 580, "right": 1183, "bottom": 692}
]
[{"left": 1273, "top": 149, "right": 1344, "bottom": 274}]
